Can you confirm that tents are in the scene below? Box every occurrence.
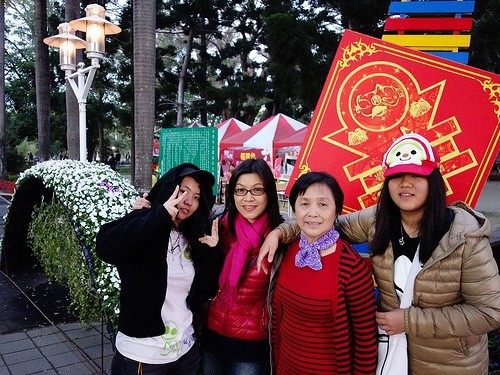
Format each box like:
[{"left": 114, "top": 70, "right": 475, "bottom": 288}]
[{"left": 187, "top": 112, "right": 308, "bottom": 158}]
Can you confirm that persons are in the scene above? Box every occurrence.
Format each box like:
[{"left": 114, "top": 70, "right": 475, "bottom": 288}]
[
  {"left": 95, "top": 158, "right": 378, "bottom": 375},
  {"left": 256, "top": 133, "right": 500, "bottom": 375}
]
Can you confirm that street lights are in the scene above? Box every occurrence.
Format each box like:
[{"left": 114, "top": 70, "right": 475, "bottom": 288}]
[{"left": 43, "top": 4, "right": 122, "bottom": 160}]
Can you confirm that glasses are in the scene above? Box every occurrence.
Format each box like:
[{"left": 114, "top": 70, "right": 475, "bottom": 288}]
[{"left": 233, "top": 187, "right": 266, "bottom": 197}]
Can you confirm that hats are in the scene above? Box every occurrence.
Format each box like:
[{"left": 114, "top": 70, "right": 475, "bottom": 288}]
[
  {"left": 380, "top": 132, "right": 441, "bottom": 176},
  {"left": 176, "top": 167, "right": 216, "bottom": 191}
]
